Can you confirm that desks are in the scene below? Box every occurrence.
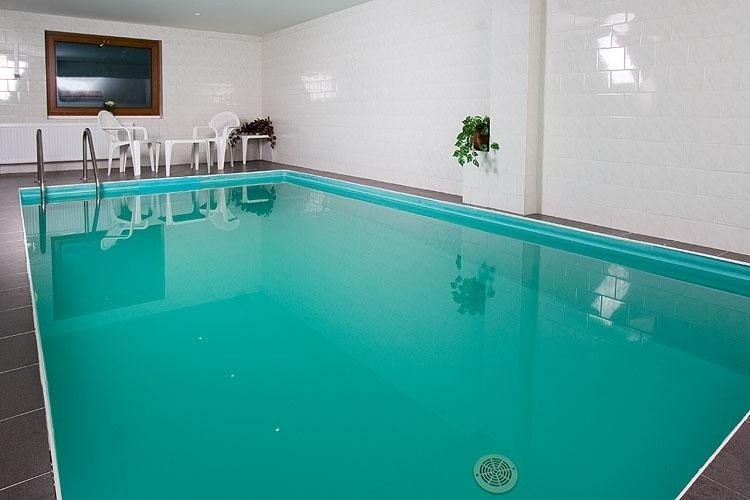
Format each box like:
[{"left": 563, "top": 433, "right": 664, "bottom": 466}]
[
  {"left": 155, "top": 139, "right": 210, "bottom": 177},
  {"left": 230, "top": 133, "right": 276, "bottom": 168},
  {"left": 240, "top": 189, "right": 270, "bottom": 205}
]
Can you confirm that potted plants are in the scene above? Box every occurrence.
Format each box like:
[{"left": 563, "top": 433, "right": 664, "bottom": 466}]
[{"left": 453, "top": 115, "right": 499, "bottom": 167}]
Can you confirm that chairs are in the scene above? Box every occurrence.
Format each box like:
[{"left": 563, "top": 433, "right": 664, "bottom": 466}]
[
  {"left": 98, "top": 110, "right": 154, "bottom": 176},
  {"left": 100, "top": 189, "right": 244, "bottom": 251},
  {"left": 192, "top": 112, "right": 240, "bottom": 177}
]
